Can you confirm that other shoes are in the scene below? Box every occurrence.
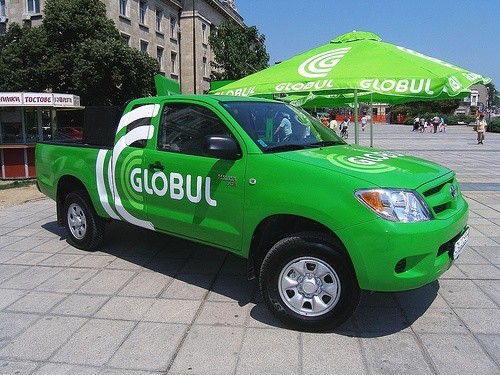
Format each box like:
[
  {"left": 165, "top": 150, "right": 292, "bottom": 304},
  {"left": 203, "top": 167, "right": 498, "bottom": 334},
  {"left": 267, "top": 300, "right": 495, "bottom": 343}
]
[
  {"left": 478, "top": 142, "right": 481, "bottom": 144},
  {"left": 481, "top": 141, "right": 483, "bottom": 144}
]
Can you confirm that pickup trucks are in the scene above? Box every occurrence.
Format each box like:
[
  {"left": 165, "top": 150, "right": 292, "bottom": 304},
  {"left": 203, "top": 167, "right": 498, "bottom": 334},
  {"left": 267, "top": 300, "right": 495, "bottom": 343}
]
[{"left": 34, "top": 72, "right": 471, "bottom": 334}]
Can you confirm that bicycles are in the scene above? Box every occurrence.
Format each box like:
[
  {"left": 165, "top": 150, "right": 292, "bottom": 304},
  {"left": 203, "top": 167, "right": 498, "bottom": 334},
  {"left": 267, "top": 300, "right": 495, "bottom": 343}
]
[{"left": 339, "top": 125, "right": 348, "bottom": 140}]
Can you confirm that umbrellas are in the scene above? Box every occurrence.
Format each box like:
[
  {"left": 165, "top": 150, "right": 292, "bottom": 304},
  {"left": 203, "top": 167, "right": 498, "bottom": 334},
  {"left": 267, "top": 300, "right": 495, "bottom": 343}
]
[{"left": 209, "top": 30, "right": 492, "bottom": 148}]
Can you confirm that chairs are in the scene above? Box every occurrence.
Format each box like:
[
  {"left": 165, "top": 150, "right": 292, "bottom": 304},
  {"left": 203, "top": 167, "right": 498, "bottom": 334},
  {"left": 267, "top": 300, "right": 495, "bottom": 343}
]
[
  {"left": 467, "top": 122, "right": 477, "bottom": 126},
  {"left": 458, "top": 121, "right": 467, "bottom": 125},
  {"left": 170, "top": 136, "right": 207, "bottom": 155}
]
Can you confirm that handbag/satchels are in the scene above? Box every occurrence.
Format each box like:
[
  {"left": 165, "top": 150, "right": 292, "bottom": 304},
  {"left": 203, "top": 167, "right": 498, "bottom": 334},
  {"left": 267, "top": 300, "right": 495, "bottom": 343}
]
[{"left": 339, "top": 124, "right": 341, "bottom": 130}]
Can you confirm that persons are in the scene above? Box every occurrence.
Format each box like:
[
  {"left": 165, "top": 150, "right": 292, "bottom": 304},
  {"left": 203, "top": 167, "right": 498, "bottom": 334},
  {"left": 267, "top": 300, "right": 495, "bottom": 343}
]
[
  {"left": 476, "top": 115, "right": 487, "bottom": 144},
  {"left": 274, "top": 113, "right": 310, "bottom": 141},
  {"left": 329, "top": 115, "right": 338, "bottom": 133},
  {"left": 361, "top": 115, "right": 367, "bottom": 131},
  {"left": 340, "top": 118, "right": 348, "bottom": 137},
  {"left": 412, "top": 115, "right": 447, "bottom": 133},
  {"left": 312, "top": 112, "right": 352, "bottom": 128}
]
